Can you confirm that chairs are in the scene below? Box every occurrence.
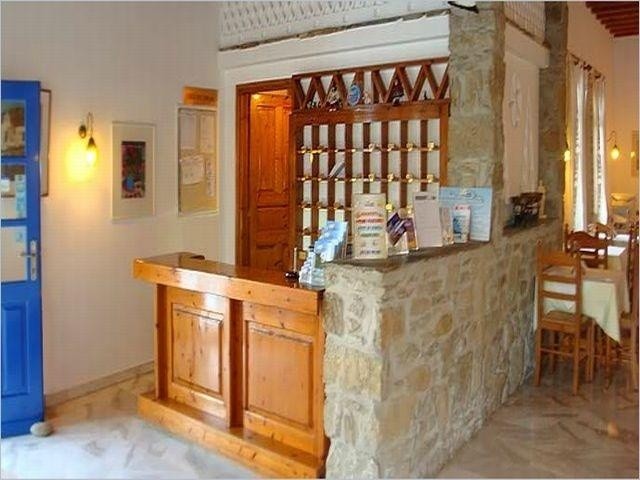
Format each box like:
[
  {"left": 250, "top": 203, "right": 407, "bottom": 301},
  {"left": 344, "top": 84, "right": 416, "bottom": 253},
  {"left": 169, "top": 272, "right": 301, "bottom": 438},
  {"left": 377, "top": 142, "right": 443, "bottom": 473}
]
[{"left": 531, "top": 193, "right": 639, "bottom": 395}]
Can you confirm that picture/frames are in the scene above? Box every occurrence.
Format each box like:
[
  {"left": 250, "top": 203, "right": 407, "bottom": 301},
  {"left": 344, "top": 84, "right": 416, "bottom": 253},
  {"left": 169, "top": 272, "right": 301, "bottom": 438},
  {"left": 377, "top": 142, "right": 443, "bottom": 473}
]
[
  {"left": 110, "top": 119, "right": 158, "bottom": 220},
  {"left": 0, "top": 87, "right": 52, "bottom": 199}
]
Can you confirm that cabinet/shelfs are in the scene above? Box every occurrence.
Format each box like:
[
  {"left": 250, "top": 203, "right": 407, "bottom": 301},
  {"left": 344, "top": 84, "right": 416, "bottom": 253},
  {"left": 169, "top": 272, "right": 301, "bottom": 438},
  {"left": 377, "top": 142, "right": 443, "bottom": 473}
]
[{"left": 288, "top": 99, "right": 451, "bottom": 236}]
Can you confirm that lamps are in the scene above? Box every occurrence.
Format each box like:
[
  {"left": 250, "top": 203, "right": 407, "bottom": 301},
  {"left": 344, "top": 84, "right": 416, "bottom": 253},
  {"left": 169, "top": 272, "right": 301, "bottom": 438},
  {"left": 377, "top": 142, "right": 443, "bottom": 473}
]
[
  {"left": 79, "top": 112, "right": 99, "bottom": 165},
  {"left": 606, "top": 130, "right": 620, "bottom": 159}
]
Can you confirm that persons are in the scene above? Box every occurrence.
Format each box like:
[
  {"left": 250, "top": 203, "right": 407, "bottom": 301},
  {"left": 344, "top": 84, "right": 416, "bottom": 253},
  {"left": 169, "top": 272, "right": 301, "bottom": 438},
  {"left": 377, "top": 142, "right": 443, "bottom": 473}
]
[
  {"left": 327, "top": 86, "right": 339, "bottom": 112},
  {"left": 537, "top": 179, "right": 546, "bottom": 215}
]
